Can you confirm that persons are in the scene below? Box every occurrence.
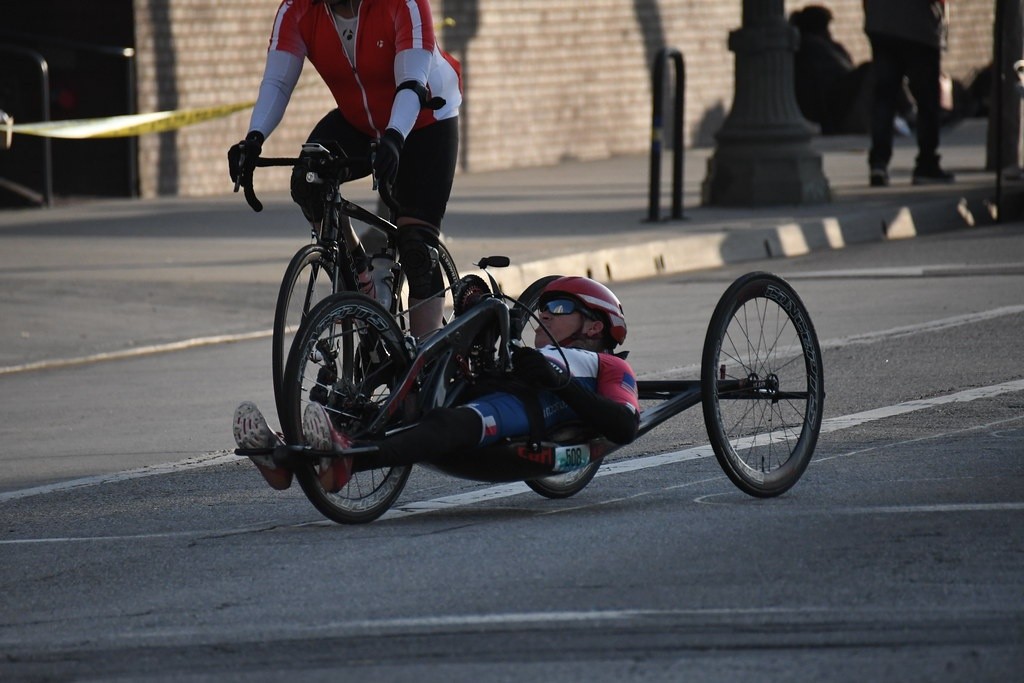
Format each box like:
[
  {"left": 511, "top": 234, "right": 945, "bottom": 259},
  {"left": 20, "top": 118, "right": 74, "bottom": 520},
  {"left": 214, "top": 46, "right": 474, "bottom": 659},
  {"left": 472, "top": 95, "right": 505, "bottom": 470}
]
[
  {"left": 233, "top": 273, "right": 645, "bottom": 490},
  {"left": 227, "top": 0, "right": 462, "bottom": 367},
  {"left": 785, "top": 4, "right": 919, "bottom": 135},
  {"left": 861, "top": 0, "right": 954, "bottom": 184}
]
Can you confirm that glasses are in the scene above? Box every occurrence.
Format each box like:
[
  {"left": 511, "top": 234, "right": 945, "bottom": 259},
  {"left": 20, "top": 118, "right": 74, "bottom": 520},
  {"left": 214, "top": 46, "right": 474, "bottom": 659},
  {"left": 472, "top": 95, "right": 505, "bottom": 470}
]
[{"left": 535, "top": 298, "right": 595, "bottom": 320}]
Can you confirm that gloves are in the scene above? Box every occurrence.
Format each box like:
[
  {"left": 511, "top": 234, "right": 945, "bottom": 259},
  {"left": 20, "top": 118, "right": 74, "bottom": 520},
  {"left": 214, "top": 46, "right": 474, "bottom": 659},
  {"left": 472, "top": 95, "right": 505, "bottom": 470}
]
[
  {"left": 509, "top": 346, "right": 562, "bottom": 391},
  {"left": 371, "top": 131, "right": 405, "bottom": 191},
  {"left": 226, "top": 132, "right": 262, "bottom": 189}
]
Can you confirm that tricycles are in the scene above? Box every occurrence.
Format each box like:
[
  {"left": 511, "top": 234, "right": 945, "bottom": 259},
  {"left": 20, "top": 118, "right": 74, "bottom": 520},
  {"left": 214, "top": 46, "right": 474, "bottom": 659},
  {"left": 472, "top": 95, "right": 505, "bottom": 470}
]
[{"left": 230, "top": 247, "right": 828, "bottom": 533}]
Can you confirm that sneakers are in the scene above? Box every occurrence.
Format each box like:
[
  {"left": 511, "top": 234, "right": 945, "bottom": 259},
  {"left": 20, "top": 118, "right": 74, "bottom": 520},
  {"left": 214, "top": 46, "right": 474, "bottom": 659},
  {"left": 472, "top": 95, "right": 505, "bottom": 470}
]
[
  {"left": 231, "top": 400, "right": 293, "bottom": 492},
  {"left": 303, "top": 400, "right": 355, "bottom": 493}
]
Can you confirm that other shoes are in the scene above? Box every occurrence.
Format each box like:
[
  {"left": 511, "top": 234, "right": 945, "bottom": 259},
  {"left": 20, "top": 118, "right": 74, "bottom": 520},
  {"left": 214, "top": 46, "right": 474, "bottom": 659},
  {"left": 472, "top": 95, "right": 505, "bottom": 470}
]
[
  {"left": 869, "top": 162, "right": 890, "bottom": 186},
  {"left": 912, "top": 166, "right": 955, "bottom": 185}
]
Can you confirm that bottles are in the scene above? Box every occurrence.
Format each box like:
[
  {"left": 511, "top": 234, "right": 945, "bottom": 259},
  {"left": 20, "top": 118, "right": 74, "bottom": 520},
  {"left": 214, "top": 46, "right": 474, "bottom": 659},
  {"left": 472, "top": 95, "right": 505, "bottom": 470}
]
[
  {"left": 354, "top": 258, "right": 377, "bottom": 300},
  {"left": 370, "top": 247, "right": 395, "bottom": 313}
]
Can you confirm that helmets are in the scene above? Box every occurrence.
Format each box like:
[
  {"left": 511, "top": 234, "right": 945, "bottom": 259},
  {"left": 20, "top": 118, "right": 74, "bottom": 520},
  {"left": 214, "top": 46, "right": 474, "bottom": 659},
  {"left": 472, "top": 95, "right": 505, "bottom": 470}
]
[{"left": 542, "top": 274, "right": 627, "bottom": 345}]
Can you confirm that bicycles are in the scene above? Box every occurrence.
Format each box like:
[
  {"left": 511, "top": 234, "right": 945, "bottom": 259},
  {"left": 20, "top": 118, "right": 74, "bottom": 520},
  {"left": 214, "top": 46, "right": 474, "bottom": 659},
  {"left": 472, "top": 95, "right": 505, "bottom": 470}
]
[{"left": 226, "top": 132, "right": 468, "bottom": 438}]
